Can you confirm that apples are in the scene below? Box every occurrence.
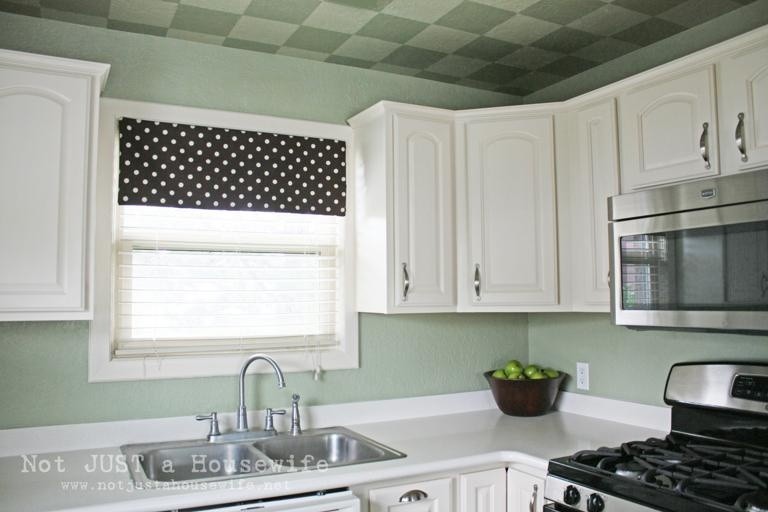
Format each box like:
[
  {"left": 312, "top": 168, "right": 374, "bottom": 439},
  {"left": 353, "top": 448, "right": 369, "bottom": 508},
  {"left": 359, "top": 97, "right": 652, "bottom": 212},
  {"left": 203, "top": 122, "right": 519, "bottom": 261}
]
[{"left": 493, "top": 361, "right": 559, "bottom": 380}]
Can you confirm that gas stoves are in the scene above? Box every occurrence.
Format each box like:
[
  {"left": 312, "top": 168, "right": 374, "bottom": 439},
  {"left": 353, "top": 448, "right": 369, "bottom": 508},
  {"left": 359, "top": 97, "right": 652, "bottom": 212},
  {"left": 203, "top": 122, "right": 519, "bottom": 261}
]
[{"left": 546, "top": 431, "right": 767, "bottom": 510}]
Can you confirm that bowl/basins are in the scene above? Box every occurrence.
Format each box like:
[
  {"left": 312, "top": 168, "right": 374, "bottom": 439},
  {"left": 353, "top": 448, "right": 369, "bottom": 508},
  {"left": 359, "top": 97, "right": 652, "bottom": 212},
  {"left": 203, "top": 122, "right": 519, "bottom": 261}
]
[{"left": 482, "top": 368, "right": 567, "bottom": 416}]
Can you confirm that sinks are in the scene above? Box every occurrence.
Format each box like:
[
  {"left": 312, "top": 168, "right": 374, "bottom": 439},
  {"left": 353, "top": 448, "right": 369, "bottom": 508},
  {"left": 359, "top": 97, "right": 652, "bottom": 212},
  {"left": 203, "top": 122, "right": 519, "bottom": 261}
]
[
  {"left": 119, "top": 434, "right": 272, "bottom": 488},
  {"left": 251, "top": 426, "right": 408, "bottom": 473}
]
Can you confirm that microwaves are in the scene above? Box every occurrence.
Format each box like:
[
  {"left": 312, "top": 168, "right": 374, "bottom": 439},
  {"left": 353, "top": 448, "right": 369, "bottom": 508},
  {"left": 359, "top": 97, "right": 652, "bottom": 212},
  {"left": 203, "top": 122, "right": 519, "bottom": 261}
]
[{"left": 606, "top": 164, "right": 768, "bottom": 338}]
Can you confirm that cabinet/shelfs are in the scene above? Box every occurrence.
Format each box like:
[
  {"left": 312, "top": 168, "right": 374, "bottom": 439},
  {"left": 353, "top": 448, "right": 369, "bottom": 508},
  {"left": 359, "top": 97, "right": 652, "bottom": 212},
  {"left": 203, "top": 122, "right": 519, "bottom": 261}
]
[
  {"left": 506, "top": 467, "right": 546, "bottom": 512},
  {"left": 0, "top": 61, "right": 100, "bottom": 322},
  {"left": 458, "top": 467, "right": 507, "bottom": 512},
  {"left": 366, "top": 475, "right": 456, "bottom": 512},
  {"left": 455, "top": 107, "right": 566, "bottom": 313},
  {"left": 565, "top": 92, "right": 621, "bottom": 314},
  {"left": 618, "top": 39, "right": 767, "bottom": 195},
  {"left": 346, "top": 113, "right": 455, "bottom": 315}
]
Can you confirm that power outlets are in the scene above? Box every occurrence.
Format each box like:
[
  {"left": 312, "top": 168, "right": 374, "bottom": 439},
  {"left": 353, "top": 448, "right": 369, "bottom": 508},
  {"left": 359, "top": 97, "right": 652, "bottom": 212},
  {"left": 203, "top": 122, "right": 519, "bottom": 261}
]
[{"left": 575, "top": 362, "right": 589, "bottom": 390}]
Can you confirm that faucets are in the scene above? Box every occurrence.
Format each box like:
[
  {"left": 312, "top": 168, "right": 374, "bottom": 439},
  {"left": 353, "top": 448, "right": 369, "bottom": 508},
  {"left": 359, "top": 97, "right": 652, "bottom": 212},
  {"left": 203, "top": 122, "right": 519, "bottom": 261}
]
[{"left": 235, "top": 353, "right": 287, "bottom": 432}]
